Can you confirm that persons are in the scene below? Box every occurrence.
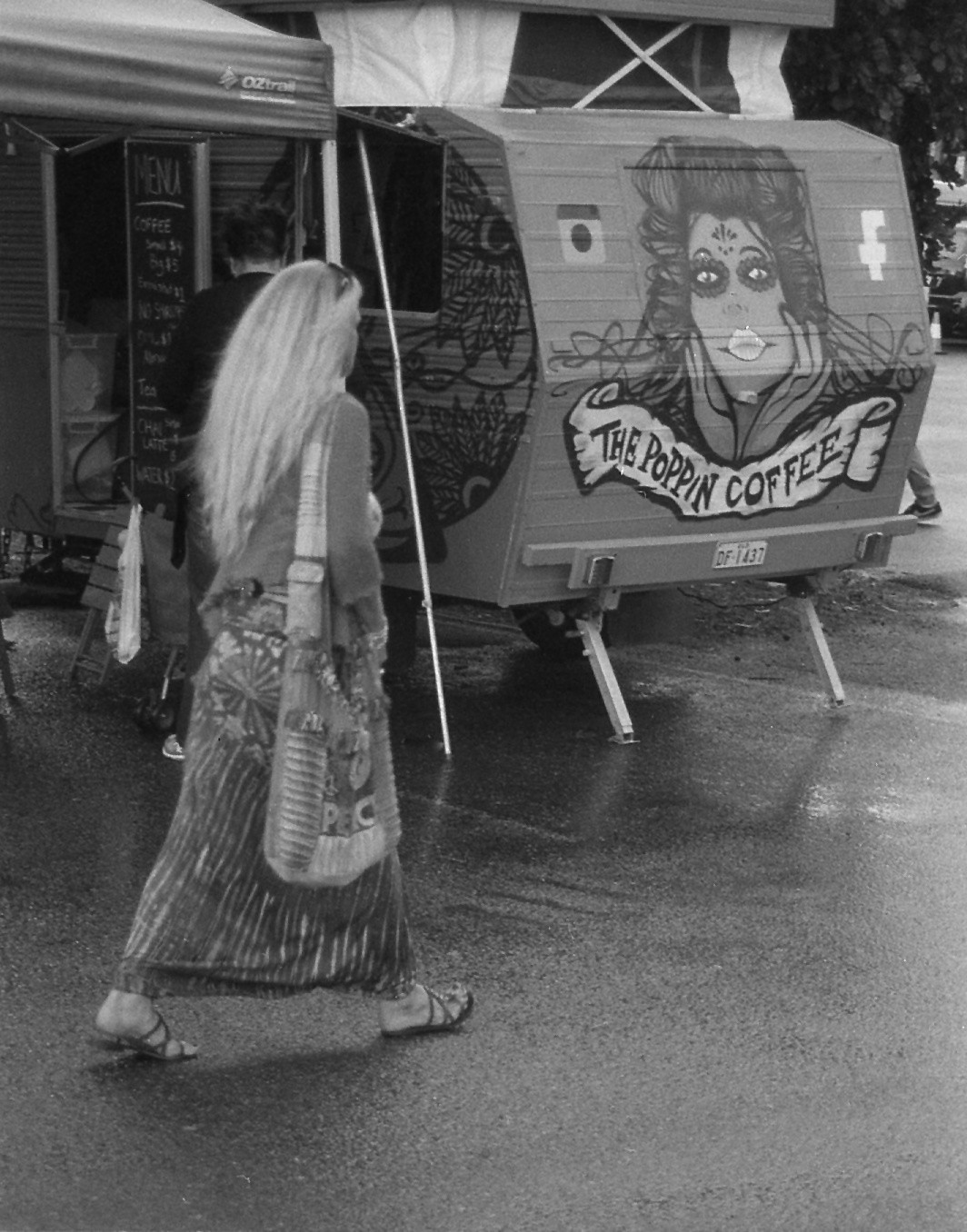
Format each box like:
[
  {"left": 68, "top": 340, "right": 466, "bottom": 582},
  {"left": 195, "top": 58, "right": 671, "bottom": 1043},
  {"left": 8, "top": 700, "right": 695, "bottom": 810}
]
[
  {"left": 95, "top": 258, "right": 476, "bottom": 1061},
  {"left": 902, "top": 444, "right": 942, "bottom": 520},
  {"left": 157, "top": 199, "right": 289, "bottom": 757}
]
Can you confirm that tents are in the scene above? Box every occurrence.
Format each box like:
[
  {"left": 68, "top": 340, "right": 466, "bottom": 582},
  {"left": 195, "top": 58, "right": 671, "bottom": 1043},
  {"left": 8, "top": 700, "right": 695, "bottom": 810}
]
[{"left": 0, "top": 0, "right": 342, "bottom": 265}]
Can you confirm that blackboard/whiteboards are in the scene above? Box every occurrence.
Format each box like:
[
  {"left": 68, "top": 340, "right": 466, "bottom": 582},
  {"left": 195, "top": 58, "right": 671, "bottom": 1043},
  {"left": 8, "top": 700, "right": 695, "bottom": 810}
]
[{"left": 120, "top": 143, "right": 212, "bottom": 509}]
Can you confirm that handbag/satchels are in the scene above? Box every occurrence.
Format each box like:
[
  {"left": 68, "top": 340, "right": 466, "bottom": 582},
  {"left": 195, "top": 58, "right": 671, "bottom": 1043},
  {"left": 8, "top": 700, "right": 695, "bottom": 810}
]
[
  {"left": 104, "top": 503, "right": 143, "bottom": 666},
  {"left": 261, "top": 641, "right": 404, "bottom": 888}
]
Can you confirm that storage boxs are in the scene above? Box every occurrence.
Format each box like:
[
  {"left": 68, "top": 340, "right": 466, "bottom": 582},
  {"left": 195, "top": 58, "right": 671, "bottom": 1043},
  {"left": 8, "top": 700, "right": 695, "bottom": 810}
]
[
  {"left": 58, "top": 332, "right": 117, "bottom": 413},
  {"left": 62, "top": 414, "right": 120, "bottom": 501}
]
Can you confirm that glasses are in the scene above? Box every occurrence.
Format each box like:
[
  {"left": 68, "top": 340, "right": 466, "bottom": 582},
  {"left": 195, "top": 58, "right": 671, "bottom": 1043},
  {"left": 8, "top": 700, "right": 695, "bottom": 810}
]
[{"left": 329, "top": 261, "right": 354, "bottom": 300}]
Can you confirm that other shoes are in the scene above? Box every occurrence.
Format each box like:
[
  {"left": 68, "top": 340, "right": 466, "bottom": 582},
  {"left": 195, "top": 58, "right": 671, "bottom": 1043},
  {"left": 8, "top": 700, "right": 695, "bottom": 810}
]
[
  {"left": 163, "top": 732, "right": 187, "bottom": 759},
  {"left": 905, "top": 501, "right": 943, "bottom": 521}
]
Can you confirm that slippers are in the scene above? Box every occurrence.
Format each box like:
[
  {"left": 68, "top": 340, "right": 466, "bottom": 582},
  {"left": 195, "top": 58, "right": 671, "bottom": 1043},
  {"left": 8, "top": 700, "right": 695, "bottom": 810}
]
[
  {"left": 83, "top": 1009, "right": 197, "bottom": 1063},
  {"left": 381, "top": 983, "right": 474, "bottom": 1035}
]
